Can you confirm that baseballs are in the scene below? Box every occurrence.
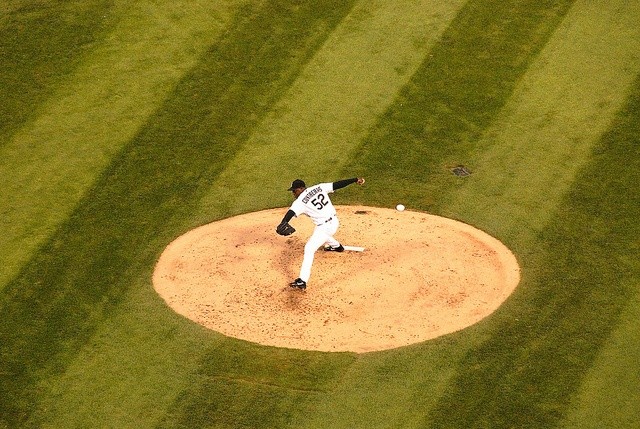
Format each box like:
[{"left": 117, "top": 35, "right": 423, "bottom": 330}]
[{"left": 396, "top": 204, "right": 405, "bottom": 211}]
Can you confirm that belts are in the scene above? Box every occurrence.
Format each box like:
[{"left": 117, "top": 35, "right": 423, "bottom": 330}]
[{"left": 317, "top": 214, "right": 336, "bottom": 227}]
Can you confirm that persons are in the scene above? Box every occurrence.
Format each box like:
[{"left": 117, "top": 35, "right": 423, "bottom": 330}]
[{"left": 276, "top": 177, "right": 365, "bottom": 290}]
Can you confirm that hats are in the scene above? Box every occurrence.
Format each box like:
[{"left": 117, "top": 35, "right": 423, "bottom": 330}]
[{"left": 286, "top": 178, "right": 306, "bottom": 192}]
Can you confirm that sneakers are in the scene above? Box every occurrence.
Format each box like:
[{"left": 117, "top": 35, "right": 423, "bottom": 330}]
[
  {"left": 287, "top": 277, "right": 307, "bottom": 289},
  {"left": 323, "top": 243, "right": 345, "bottom": 253}
]
